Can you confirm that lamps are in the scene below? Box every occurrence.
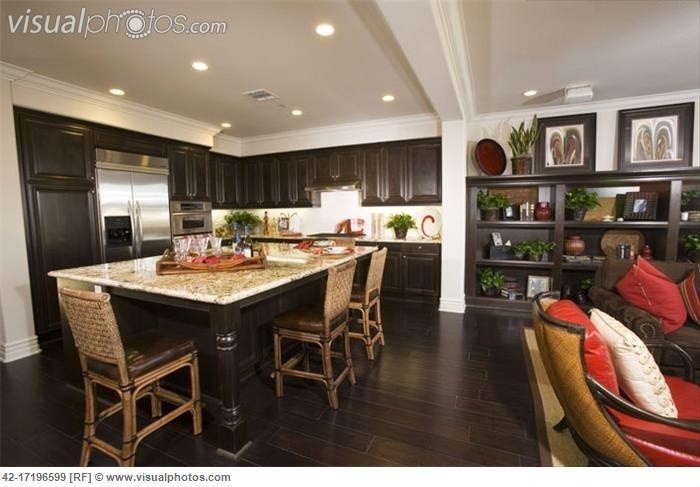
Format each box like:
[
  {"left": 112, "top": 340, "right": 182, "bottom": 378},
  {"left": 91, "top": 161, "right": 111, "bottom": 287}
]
[{"left": 565, "top": 83, "right": 593, "bottom": 102}]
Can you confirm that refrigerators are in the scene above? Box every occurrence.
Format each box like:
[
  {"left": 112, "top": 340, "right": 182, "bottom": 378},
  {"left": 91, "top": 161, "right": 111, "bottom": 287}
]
[{"left": 94, "top": 165, "right": 171, "bottom": 263}]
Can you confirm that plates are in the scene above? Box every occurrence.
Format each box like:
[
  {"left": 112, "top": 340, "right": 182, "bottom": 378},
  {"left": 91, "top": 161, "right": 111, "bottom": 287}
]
[
  {"left": 416, "top": 208, "right": 442, "bottom": 239},
  {"left": 313, "top": 240, "right": 331, "bottom": 247},
  {"left": 324, "top": 246, "right": 353, "bottom": 255},
  {"left": 474, "top": 139, "right": 506, "bottom": 176}
]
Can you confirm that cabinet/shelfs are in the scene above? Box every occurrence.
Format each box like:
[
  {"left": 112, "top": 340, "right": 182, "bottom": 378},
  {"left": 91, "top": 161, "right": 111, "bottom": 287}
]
[
  {"left": 466, "top": 167, "right": 700, "bottom": 320},
  {"left": 13, "top": 105, "right": 102, "bottom": 336},
  {"left": 355, "top": 241, "right": 441, "bottom": 302},
  {"left": 166, "top": 138, "right": 442, "bottom": 209}
]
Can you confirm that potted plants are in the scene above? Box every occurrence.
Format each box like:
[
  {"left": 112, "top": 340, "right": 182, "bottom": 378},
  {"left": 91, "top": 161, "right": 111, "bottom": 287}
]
[
  {"left": 386, "top": 213, "right": 417, "bottom": 239},
  {"left": 508, "top": 113, "right": 543, "bottom": 173},
  {"left": 225, "top": 211, "right": 261, "bottom": 234},
  {"left": 679, "top": 231, "right": 700, "bottom": 262},
  {"left": 681, "top": 190, "right": 700, "bottom": 211},
  {"left": 477, "top": 190, "right": 558, "bottom": 297},
  {"left": 565, "top": 186, "right": 598, "bottom": 221}
]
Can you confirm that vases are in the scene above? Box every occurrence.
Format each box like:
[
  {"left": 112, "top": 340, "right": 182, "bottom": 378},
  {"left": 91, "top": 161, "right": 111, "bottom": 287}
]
[{"left": 565, "top": 236, "right": 586, "bottom": 256}]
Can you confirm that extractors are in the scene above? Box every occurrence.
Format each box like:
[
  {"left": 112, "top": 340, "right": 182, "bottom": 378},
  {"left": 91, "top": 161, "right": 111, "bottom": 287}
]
[{"left": 303, "top": 181, "right": 360, "bottom": 191}]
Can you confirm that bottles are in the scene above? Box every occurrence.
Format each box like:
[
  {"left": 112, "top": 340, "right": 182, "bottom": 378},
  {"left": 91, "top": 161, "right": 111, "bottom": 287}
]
[
  {"left": 263, "top": 212, "right": 269, "bottom": 235},
  {"left": 232, "top": 223, "right": 242, "bottom": 255},
  {"left": 243, "top": 223, "right": 253, "bottom": 258},
  {"left": 271, "top": 218, "right": 277, "bottom": 235}
]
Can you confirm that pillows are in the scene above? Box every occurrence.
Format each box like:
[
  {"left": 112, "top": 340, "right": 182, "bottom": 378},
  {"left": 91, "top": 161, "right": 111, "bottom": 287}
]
[{"left": 545, "top": 255, "right": 700, "bottom": 420}]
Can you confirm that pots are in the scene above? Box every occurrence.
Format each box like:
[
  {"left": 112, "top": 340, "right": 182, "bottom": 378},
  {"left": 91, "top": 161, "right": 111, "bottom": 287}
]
[{"left": 338, "top": 218, "right": 364, "bottom": 235}]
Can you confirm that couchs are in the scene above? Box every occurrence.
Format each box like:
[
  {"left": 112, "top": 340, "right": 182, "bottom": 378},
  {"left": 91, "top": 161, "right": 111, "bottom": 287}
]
[{"left": 588, "top": 257, "right": 699, "bottom": 385}]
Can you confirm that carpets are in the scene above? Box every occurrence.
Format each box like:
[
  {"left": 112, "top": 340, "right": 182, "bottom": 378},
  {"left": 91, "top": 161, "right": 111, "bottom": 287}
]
[{"left": 516, "top": 324, "right": 589, "bottom": 466}]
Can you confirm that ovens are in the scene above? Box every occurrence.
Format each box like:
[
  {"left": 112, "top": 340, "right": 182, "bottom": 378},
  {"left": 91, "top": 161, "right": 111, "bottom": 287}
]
[{"left": 171, "top": 212, "right": 212, "bottom": 236}]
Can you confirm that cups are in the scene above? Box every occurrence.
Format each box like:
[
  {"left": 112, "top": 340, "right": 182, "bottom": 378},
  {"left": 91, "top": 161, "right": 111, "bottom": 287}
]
[{"left": 173, "top": 237, "right": 223, "bottom": 261}]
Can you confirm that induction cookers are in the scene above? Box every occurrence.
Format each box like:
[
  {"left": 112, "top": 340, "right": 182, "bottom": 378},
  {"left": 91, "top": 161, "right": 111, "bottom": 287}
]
[{"left": 306, "top": 232, "right": 367, "bottom": 238}]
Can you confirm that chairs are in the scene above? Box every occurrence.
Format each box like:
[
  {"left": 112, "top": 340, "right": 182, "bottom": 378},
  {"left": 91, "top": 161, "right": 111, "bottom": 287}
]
[
  {"left": 348, "top": 246, "right": 387, "bottom": 359},
  {"left": 271, "top": 258, "right": 357, "bottom": 410},
  {"left": 532, "top": 290, "right": 700, "bottom": 466},
  {"left": 57, "top": 287, "right": 202, "bottom": 466}
]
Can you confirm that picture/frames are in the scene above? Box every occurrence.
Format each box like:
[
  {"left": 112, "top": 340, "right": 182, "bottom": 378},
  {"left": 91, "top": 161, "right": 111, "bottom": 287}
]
[
  {"left": 534, "top": 112, "right": 597, "bottom": 175},
  {"left": 618, "top": 102, "right": 694, "bottom": 169},
  {"left": 623, "top": 192, "right": 658, "bottom": 219},
  {"left": 525, "top": 274, "right": 552, "bottom": 299}
]
